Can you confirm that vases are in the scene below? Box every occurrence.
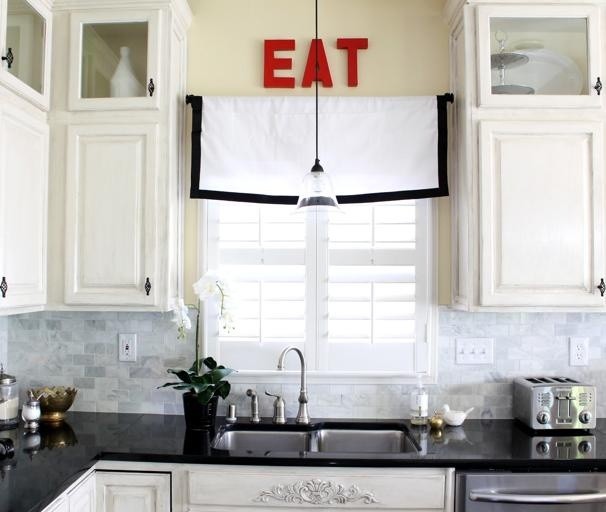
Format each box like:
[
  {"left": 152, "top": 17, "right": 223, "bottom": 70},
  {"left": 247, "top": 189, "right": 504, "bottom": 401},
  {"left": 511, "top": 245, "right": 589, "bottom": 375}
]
[{"left": 178, "top": 384, "right": 221, "bottom": 458}]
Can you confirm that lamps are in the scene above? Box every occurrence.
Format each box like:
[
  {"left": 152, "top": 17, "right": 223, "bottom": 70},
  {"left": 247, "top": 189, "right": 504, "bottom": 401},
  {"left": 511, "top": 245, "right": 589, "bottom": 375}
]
[{"left": 286, "top": 1, "right": 345, "bottom": 209}]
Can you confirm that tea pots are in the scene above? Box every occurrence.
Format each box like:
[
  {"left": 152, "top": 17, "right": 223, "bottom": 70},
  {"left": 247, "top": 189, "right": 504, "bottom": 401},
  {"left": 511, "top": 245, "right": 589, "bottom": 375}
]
[
  {"left": 22, "top": 393, "right": 45, "bottom": 428},
  {"left": 21, "top": 430, "right": 42, "bottom": 460}
]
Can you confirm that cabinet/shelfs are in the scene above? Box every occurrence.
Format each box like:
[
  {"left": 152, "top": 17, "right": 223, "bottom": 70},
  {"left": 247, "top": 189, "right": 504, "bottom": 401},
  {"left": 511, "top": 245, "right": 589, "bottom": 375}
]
[
  {"left": 449, "top": 112, "right": 606, "bottom": 313},
  {"left": 178, "top": 465, "right": 455, "bottom": 512},
  {"left": 44, "top": 458, "right": 173, "bottom": 511},
  {"left": 1, "top": 0, "right": 52, "bottom": 315},
  {"left": 451, "top": 5, "right": 606, "bottom": 105},
  {"left": 49, "top": 0, "right": 187, "bottom": 312}
]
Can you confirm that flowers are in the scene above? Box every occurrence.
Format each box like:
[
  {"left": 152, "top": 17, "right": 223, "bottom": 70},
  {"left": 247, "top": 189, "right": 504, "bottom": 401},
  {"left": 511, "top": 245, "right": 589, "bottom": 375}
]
[{"left": 157, "top": 266, "right": 240, "bottom": 402}]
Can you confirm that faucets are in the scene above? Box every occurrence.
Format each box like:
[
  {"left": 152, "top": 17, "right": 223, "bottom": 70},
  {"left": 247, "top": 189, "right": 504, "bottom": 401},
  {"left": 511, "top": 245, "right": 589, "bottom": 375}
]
[
  {"left": 246, "top": 388, "right": 262, "bottom": 422},
  {"left": 276, "top": 346, "right": 311, "bottom": 425}
]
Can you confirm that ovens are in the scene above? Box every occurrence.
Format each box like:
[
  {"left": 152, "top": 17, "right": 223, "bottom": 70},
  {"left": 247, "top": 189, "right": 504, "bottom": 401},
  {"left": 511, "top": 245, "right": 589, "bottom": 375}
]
[{"left": 462, "top": 471, "right": 605, "bottom": 510}]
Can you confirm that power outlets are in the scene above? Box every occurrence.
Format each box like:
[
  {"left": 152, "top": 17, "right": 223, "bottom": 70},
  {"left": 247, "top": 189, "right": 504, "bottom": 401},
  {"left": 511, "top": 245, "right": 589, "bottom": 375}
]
[{"left": 569, "top": 335, "right": 589, "bottom": 367}]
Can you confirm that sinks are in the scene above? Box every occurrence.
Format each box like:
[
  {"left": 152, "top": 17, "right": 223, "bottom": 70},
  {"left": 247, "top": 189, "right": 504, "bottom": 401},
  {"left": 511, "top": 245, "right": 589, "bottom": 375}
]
[
  {"left": 315, "top": 428, "right": 419, "bottom": 454},
  {"left": 217, "top": 431, "right": 314, "bottom": 454}
]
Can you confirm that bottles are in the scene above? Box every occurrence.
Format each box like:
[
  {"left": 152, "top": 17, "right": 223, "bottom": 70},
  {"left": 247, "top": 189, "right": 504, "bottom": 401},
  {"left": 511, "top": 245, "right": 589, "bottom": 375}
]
[
  {"left": 410, "top": 371, "right": 429, "bottom": 426},
  {"left": 413, "top": 430, "right": 428, "bottom": 456}
]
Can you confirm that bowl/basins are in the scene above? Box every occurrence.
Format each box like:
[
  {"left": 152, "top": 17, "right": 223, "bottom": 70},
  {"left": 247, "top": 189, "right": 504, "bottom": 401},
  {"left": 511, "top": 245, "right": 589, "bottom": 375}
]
[
  {"left": 446, "top": 427, "right": 464, "bottom": 441},
  {"left": 38, "top": 422, "right": 79, "bottom": 448},
  {"left": 27, "top": 386, "right": 77, "bottom": 421},
  {"left": 445, "top": 410, "right": 465, "bottom": 426}
]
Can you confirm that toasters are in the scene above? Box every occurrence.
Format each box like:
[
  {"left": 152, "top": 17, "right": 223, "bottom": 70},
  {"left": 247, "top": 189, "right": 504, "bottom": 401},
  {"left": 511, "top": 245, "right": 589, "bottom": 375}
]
[
  {"left": 513, "top": 375, "right": 594, "bottom": 431},
  {"left": 511, "top": 429, "right": 597, "bottom": 459}
]
